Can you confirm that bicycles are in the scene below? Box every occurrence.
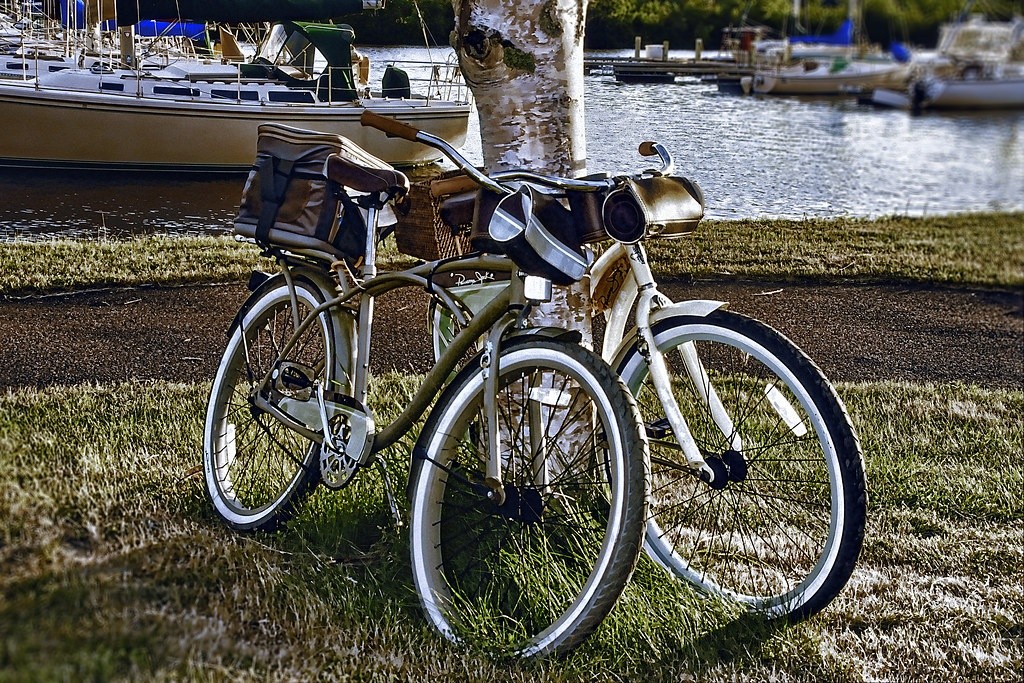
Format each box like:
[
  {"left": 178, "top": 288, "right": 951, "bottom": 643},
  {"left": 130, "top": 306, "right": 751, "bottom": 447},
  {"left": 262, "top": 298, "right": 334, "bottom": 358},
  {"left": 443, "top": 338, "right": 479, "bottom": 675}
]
[{"left": 200, "top": 109, "right": 869, "bottom": 665}]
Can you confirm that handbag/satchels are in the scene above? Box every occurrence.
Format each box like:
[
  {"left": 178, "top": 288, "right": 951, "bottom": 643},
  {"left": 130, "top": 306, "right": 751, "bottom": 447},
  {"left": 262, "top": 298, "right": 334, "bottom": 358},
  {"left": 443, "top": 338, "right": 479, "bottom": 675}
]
[{"left": 234, "top": 122, "right": 411, "bottom": 271}]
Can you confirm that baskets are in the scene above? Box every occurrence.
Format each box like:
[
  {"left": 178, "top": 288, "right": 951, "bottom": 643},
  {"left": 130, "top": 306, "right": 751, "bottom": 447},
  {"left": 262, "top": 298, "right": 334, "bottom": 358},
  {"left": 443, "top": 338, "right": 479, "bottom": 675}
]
[{"left": 394, "top": 167, "right": 487, "bottom": 261}]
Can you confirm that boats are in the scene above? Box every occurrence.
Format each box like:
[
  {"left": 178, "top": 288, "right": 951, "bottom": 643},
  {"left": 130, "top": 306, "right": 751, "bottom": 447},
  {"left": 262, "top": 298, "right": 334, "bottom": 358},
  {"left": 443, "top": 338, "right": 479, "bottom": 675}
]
[
  {"left": 699, "top": 0, "right": 1024, "bottom": 110},
  {"left": 613, "top": 71, "right": 676, "bottom": 83},
  {"left": 0, "top": 1, "right": 478, "bottom": 174}
]
